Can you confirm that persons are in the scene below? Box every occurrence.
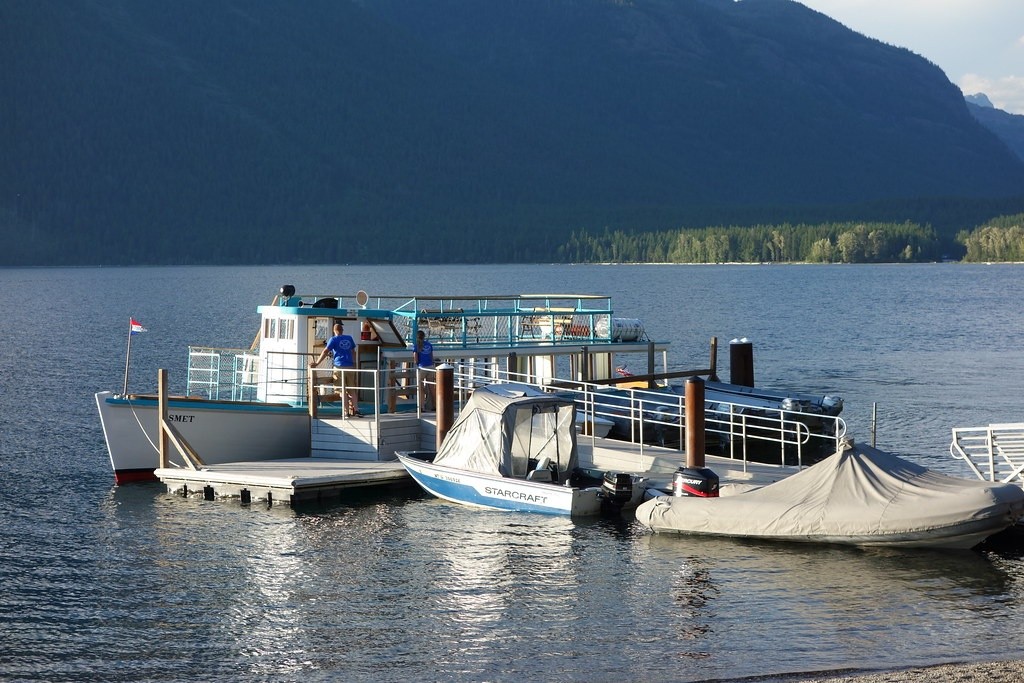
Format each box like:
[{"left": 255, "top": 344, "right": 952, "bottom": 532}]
[
  {"left": 413, "top": 330, "right": 436, "bottom": 412},
  {"left": 308, "top": 323, "right": 364, "bottom": 418}
]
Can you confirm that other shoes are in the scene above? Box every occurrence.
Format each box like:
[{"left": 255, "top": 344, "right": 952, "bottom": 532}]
[
  {"left": 430, "top": 408, "right": 436, "bottom": 412},
  {"left": 340, "top": 414, "right": 350, "bottom": 419},
  {"left": 354, "top": 411, "right": 359, "bottom": 417},
  {"left": 416, "top": 409, "right": 426, "bottom": 413}
]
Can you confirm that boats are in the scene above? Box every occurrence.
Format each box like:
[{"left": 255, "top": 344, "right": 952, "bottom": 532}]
[
  {"left": 393, "top": 379, "right": 644, "bottom": 519},
  {"left": 632, "top": 434, "right": 1023, "bottom": 554},
  {"left": 92, "top": 283, "right": 671, "bottom": 489},
  {"left": 556, "top": 341, "right": 847, "bottom": 446}
]
